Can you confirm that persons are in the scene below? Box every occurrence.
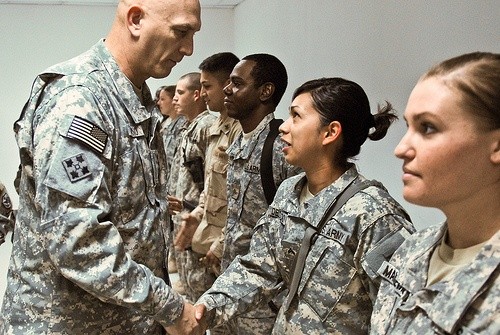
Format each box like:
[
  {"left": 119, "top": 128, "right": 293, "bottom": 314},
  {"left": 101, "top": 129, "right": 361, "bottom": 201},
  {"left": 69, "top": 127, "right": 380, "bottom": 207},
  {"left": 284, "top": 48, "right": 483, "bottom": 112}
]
[
  {"left": 155, "top": 86, "right": 167, "bottom": 109},
  {"left": 215, "top": 53, "right": 311, "bottom": 335},
  {"left": 195, "top": 77, "right": 417, "bottom": 335},
  {"left": 1, "top": 0, "right": 208, "bottom": 335},
  {"left": 154, "top": 85, "right": 178, "bottom": 195},
  {"left": 165, "top": 72, "right": 216, "bottom": 300},
  {"left": 367, "top": 50, "right": 500, "bottom": 335},
  {"left": 174, "top": 52, "right": 242, "bottom": 306}
]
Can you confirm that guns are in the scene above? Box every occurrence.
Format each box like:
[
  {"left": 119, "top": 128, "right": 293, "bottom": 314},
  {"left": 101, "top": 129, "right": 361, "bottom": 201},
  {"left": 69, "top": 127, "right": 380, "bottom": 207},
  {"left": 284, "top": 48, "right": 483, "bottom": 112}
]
[{"left": 182, "top": 156, "right": 205, "bottom": 214}]
